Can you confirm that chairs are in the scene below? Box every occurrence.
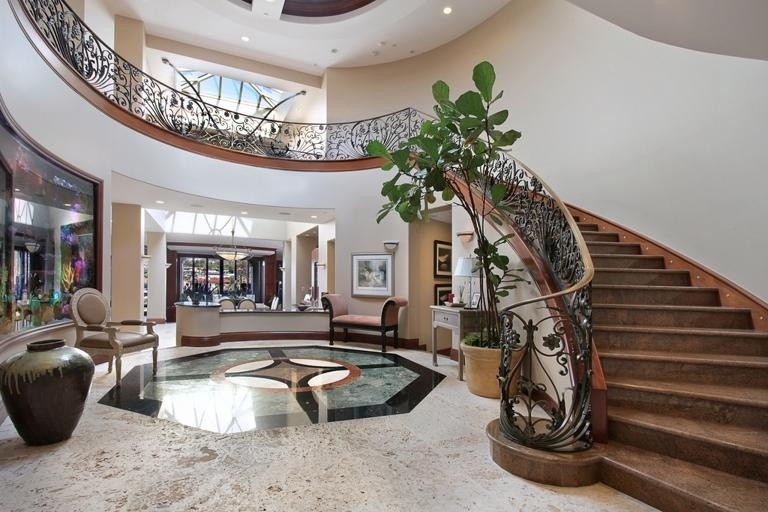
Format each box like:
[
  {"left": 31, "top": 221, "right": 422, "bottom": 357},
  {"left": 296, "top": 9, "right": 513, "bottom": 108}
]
[
  {"left": 67, "top": 288, "right": 159, "bottom": 392},
  {"left": 219, "top": 299, "right": 258, "bottom": 309},
  {"left": 270, "top": 295, "right": 280, "bottom": 310}
]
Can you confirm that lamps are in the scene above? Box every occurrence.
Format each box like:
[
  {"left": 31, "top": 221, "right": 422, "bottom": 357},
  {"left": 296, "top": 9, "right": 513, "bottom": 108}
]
[
  {"left": 453, "top": 254, "right": 486, "bottom": 310},
  {"left": 216, "top": 230, "right": 251, "bottom": 260}
]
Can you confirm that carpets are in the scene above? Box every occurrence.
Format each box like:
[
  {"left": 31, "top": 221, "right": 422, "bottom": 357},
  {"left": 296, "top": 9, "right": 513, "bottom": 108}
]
[{"left": 471, "top": 292, "right": 480, "bottom": 309}]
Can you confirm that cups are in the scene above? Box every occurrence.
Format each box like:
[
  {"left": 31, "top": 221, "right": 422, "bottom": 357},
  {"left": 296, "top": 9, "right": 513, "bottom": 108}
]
[{"left": 448, "top": 294, "right": 460, "bottom": 303}]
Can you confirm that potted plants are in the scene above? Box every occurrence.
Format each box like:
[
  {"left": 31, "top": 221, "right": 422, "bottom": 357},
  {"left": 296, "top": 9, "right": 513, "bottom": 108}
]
[
  {"left": 184, "top": 278, "right": 210, "bottom": 305},
  {"left": 364, "top": 60, "right": 528, "bottom": 399}
]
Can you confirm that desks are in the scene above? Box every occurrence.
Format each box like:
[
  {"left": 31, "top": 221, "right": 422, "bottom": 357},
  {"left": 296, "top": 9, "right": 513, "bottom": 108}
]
[{"left": 430, "top": 304, "right": 495, "bottom": 381}]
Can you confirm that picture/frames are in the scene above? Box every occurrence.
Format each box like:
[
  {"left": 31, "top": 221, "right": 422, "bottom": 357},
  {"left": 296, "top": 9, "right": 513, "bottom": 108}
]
[
  {"left": 350, "top": 253, "right": 394, "bottom": 299},
  {"left": 433, "top": 240, "right": 452, "bottom": 305}
]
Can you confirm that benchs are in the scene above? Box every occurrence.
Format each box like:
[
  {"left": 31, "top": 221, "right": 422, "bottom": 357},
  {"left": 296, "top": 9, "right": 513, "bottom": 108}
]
[{"left": 321, "top": 293, "right": 408, "bottom": 352}]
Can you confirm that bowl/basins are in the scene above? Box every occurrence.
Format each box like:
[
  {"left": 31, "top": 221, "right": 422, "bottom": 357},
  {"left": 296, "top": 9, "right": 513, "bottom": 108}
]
[
  {"left": 444, "top": 302, "right": 452, "bottom": 306},
  {"left": 296, "top": 305, "right": 311, "bottom": 311}
]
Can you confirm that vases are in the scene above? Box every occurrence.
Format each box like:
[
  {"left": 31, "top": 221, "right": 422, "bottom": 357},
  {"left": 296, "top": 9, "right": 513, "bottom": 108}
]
[{"left": 0, "top": 340, "right": 96, "bottom": 445}]
